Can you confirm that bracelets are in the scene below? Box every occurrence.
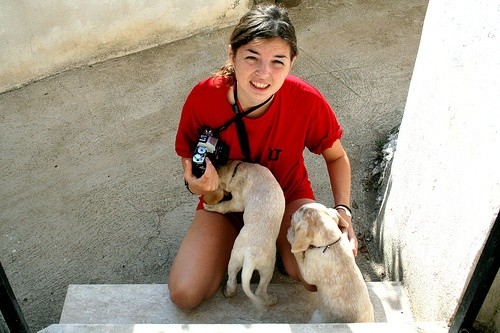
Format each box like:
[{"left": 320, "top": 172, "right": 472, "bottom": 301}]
[{"left": 333, "top": 205, "right": 352, "bottom": 218}]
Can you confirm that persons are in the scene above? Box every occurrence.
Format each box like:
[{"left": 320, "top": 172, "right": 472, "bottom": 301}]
[{"left": 167, "top": 3, "right": 358, "bottom": 311}]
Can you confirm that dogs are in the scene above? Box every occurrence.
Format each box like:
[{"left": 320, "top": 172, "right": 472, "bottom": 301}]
[
  {"left": 286, "top": 202, "right": 375, "bottom": 324},
  {"left": 203, "top": 160, "right": 285, "bottom": 308}
]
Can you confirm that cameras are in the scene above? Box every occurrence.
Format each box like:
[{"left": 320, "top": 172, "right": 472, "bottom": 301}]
[{"left": 190, "top": 125, "right": 230, "bottom": 178}]
